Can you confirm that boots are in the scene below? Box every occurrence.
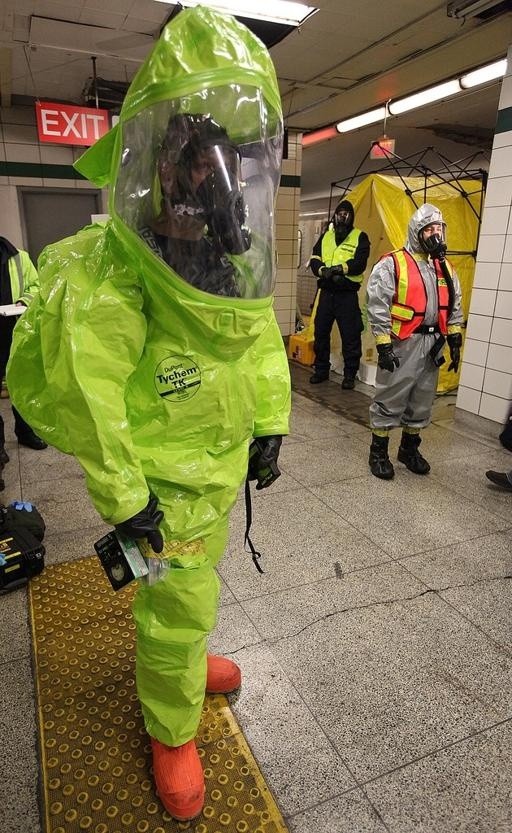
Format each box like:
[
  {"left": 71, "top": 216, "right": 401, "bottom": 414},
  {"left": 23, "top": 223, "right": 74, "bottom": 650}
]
[
  {"left": 368, "top": 433, "right": 394, "bottom": 479},
  {"left": 398, "top": 431, "right": 430, "bottom": 474}
]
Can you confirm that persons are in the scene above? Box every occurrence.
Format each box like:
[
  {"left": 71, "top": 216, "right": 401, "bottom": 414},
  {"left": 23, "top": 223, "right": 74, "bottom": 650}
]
[
  {"left": 481, "top": 466, "right": 511, "bottom": 492},
  {"left": 309, "top": 200, "right": 369, "bottom": 391},
  {"left": 366, "top": 203, "right": 463, "bottom": 481},
  {"left": 0, "top": 233, "right": 47, "bottom": 464},
  {"left": 12, "top": 112, "right": 292, "bottom": 823}
]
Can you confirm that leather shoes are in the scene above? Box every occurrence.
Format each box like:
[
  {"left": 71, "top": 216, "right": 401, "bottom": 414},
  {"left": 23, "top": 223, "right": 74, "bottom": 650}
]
[{"left": 17, "top": 434, "right": 48, "bottom": 450}]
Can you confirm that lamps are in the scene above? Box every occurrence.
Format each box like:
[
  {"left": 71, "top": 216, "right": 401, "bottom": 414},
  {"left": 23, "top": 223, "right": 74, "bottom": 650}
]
[{"left": 302, "top": 56, "right": 508, "bottom": 148}]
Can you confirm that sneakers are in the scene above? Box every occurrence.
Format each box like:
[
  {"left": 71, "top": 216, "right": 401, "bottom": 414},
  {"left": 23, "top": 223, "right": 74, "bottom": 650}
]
[
  {"left": 310, "top": 370, "right": 329, "bottom": 384},
  {"left": 150, "top": 735, "right": 205, "bottom": 822},
  {"left": 204, "top": 654, "right": 241, "bottom": 693},
  {"left": 342, "top": 376, "right": 355, "bottom": 389},
  {"left": 486, "top": 469, "right": 512, "bottom": 490}
]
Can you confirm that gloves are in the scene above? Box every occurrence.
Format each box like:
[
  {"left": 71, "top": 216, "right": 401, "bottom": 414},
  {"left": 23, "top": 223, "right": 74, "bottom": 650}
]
[
  {"left": 319, "top": 266, "right": 344, "bottom": 278},
  {"left": 248, "top": 435, "right": 282, "bottom": 490},
  {"left": 447, "top": 332, "right": 462, "bottom": 372},
  {"left": 376, "top": 342, "right": 400, "bottom": 372},
  {"left": 115, "top": 498, "right": 165, "bottom": 553}
]
